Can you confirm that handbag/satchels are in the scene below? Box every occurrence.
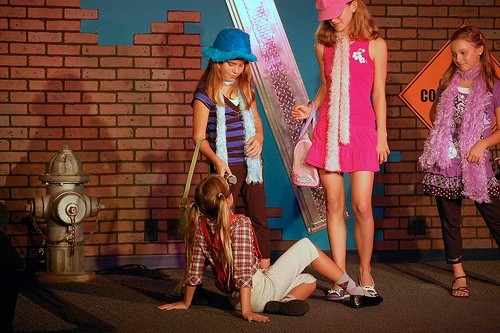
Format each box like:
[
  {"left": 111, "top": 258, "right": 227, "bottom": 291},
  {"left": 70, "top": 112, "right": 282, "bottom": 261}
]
[
  {"left": 178, "top": 202, "right": 200, "bottom": 234},
  {"left": 291, "top": 100, "right": 320, "bottom": 189}
]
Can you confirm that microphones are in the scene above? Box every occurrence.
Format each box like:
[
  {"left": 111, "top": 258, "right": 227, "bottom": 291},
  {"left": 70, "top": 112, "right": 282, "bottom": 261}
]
[{"left": 225, "top": 172, "right": 237, "bottom": 185}]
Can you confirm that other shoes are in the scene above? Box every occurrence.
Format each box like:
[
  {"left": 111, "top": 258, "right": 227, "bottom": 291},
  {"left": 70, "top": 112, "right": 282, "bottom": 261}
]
[
  {"left": 264, "top": 299, "right": 309, "bottom": 316},
  {"left": 349, "top": 295, "right": 383, "bottom": 308}
]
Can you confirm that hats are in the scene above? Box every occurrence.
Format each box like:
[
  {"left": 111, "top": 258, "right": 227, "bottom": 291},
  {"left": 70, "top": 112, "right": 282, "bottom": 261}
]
[
  {"left": 316, "top": 0, "right": 352, "bottom": 21},
  {"left": 201, "top": 27, "right": 257, "bottom": 63}
]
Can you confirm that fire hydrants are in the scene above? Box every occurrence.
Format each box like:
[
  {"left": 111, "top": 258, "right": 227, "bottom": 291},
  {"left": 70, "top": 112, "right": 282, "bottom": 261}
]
[{"left": 23, "top": 144, "right": 108, "bottom": 284}]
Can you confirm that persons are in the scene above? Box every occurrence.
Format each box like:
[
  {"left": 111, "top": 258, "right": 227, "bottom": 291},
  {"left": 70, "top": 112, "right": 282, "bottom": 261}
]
[
  {"left": 292, "top": 0, "right": 390, "bottom": 300},
  {"left": 157, "top": 175, "right": 383, "bottom": 323},
  {"left": 191, "top": 28, "right": 272, "bottom": 273},
  {"left": 419, "top": 25, "right": 500, "bottom": 298}
]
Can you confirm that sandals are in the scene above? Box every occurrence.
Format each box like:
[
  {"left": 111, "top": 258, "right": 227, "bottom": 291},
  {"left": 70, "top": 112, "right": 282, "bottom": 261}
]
[
  {"left": 356, "top": 273, "right": 377, "bottom": 295},
  {"left": 452, "top": 274, "right": 469, "bottom": 298},
  {"left": 326, "top": 287, "right": 350, "bottom": 300}
]
